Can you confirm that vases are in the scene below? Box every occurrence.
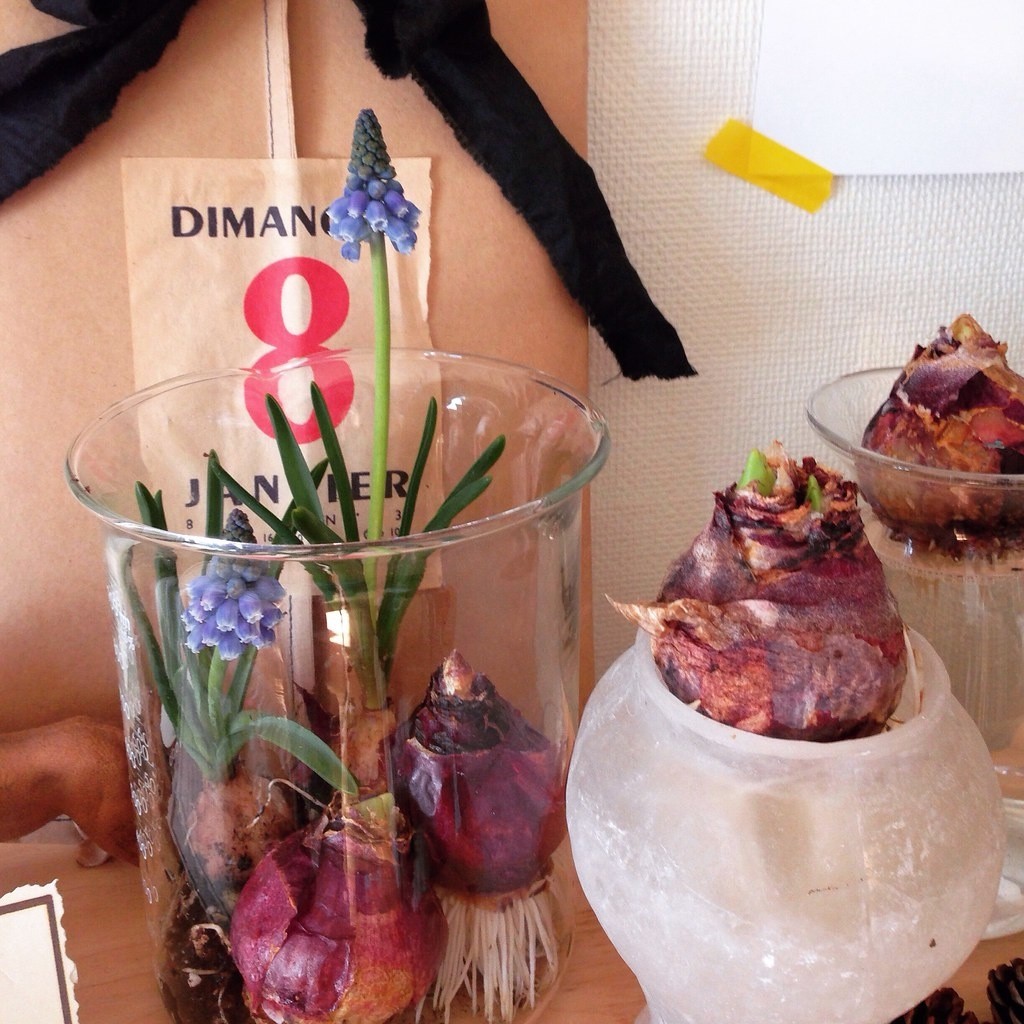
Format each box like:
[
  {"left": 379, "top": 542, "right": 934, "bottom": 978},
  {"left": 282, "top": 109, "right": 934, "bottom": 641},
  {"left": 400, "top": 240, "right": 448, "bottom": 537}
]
[{"left": 58, "top": 347, "right": 607, "bottom": 1024}]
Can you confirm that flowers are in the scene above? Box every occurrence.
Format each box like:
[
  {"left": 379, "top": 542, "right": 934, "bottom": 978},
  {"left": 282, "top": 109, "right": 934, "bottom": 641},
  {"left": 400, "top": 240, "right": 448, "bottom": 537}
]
[{"left": 131, "top": 109, "right": 505, "bottom": 793}]
